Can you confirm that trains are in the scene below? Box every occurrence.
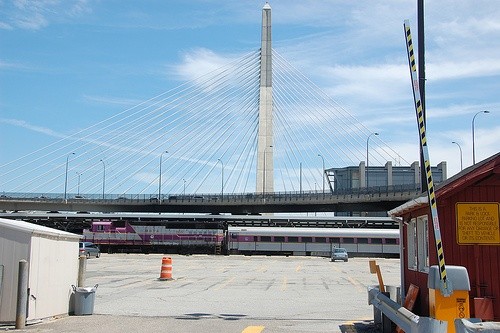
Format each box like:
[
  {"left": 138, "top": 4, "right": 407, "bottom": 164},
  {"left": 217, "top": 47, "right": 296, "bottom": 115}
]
[{"left": 0, "top": 211, "right": 401, "bottom": 259}]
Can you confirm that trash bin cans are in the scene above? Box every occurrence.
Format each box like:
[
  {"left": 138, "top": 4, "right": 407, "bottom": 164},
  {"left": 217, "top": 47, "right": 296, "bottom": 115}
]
[{"left": 72, "top": 284, "right": 99, "bottom": 316}]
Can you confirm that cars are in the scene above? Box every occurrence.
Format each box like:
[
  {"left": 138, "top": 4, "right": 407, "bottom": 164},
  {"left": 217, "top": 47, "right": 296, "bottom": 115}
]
[
  {"left": 331, "top": 247, "right": 348, "bottom": 262},
  {"left": 79, "top": 241, "right": 101, "bottom": 259}
]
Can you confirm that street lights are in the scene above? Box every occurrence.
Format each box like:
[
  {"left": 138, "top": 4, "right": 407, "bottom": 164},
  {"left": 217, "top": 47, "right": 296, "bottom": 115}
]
[
  {"left": 451, "top": 141, "right": 462, "bottom": 171},
  {"left": 472, "top": 110, "right": 490, "bottom": 164},
  {"left": 263, "top": 145, "right": 273, "bottom": 197},
  {"left": 64, "top": 153, "right": 75, "bottom": 198},
  {"left": 367, "top": 132, "right": 379, "bottom": 193},
  {"left": 100, "top": 159, "right": 105, "bottom": 199},
  {"left": 318, "top": 154, "right": 324, "bottom": 197},
  {"left": 158, "top": 150, "right": 169, "bottom": 200},
  {"left": 76, "top": 172, "right": 80, "bottom": 196},
  {"left": 218, "top": 158, "right": 223, "bottom": 202}
]
